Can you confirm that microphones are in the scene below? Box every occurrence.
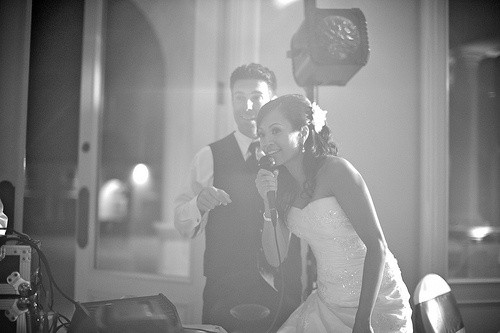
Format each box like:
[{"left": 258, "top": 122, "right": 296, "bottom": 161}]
[{"left": 260, "top": 156, "right": 277, "bottom": 228}]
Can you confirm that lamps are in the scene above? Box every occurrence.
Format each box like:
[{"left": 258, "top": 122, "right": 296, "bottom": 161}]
[{"left": 287, "top": 7, "right": 369, "bottom": 87}]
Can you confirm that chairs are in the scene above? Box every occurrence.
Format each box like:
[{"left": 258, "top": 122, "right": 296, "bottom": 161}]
[{"left": 412, "top": 273, "right": 466, "bottom": 333}]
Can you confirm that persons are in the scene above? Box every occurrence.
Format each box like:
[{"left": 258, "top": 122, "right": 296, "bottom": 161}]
[
  {"left": 174, "top": 63, "right": 315, "bottom": 333},
  {"left": 255, "top": 94, "right": 414, "bottom": 333}
]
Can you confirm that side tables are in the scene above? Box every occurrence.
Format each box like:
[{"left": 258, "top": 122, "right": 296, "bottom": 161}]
[{"left": 0, "top": 239, "right": 40, "bottom": 333}]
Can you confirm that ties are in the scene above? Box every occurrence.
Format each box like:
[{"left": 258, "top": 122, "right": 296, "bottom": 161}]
[{"left": 245, "top": 141, "right": 265, "bottom": 178}]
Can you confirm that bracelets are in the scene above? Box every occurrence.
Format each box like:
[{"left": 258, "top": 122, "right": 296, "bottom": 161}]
[{"left": 263, "top": 209, "right": 278, "bottom": 221}]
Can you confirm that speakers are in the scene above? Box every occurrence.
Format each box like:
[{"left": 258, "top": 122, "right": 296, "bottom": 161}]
[{"left": 66, "top": 292, "right": 185, "bottom": 333}]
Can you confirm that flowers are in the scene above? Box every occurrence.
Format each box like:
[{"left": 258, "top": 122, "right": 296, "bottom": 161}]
[{"left": 310, "top": 102, "right": 328, "bottom": 134}]
